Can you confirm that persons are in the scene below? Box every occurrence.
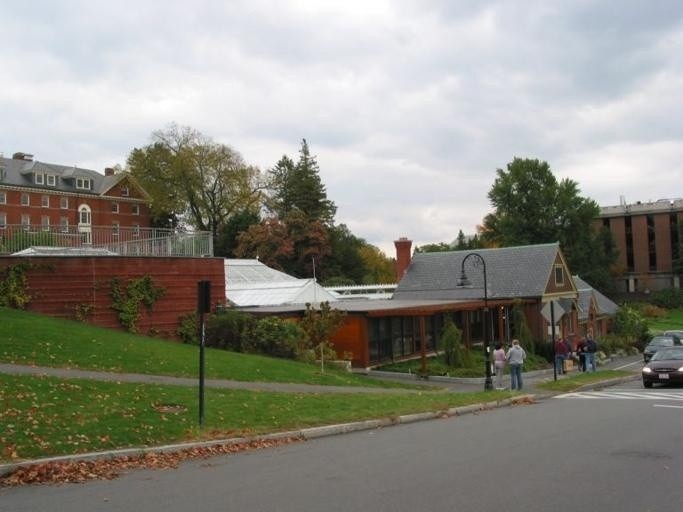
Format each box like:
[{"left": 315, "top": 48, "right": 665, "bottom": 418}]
[
  {"left": 492, "top": 339, "right": 526, "bottom": 392},
  {"left": 555, "top": 333, "right": 598, "bottom": 375}
]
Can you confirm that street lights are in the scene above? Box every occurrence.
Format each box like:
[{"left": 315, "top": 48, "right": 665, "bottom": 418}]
[{"left": 456, "top": 252, "right": 495, "bottom": 392}]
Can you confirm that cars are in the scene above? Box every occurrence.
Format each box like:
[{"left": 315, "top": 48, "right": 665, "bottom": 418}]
[{"left": 642, "top": 331, "right": 683, "bottom": 388}]
